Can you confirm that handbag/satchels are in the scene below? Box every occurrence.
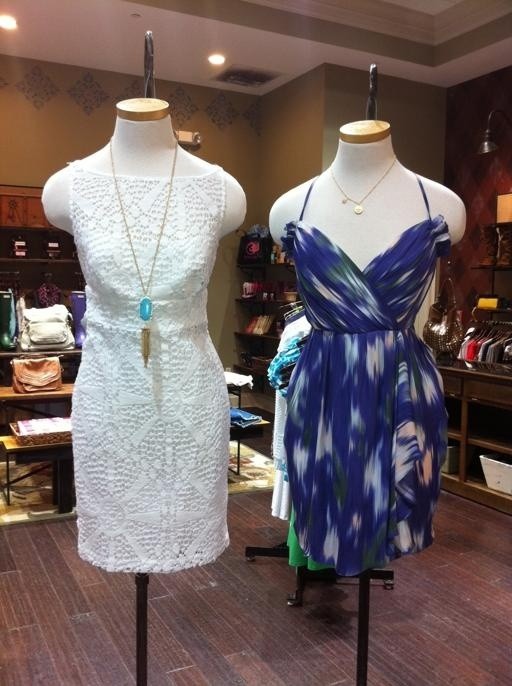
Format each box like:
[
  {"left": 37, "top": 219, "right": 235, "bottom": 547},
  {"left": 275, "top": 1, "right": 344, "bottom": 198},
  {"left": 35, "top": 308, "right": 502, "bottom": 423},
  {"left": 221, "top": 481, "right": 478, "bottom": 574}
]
[
  {"left": 235, "top": 228, "right": 272, "bottom": 264},
  {"left": 421, "top": 277, "right": 463, "bottom": 353},
  {"left": 18, "top": 304, "right": 75, "bottom": 353},
  {"left": 8, "top": 353, "right": 65, "bottom": 395}
]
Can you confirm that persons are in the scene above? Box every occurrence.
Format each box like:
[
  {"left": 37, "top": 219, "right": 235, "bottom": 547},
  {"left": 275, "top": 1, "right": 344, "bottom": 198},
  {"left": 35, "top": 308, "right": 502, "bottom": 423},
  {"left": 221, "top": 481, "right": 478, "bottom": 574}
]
[
  {"left": 264, "top": 112, "right": 471, "bottom": 584},
  {"left": 37, "top": 93, "right": 250, "bottom": 578}
]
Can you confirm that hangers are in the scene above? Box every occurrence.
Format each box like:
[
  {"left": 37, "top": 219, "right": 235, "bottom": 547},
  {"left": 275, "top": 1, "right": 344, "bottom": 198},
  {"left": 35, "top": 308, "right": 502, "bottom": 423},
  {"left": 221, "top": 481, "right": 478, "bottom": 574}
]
[{"left": 468, "top": 321, "right": 512, "bottom": 340}]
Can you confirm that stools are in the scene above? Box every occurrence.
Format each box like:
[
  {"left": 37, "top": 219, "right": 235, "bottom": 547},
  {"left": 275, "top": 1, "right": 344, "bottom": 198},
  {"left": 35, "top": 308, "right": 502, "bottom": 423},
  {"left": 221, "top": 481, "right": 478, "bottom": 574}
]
[{"left": 50, "top": 457, "right": 75, "bottom": 512}]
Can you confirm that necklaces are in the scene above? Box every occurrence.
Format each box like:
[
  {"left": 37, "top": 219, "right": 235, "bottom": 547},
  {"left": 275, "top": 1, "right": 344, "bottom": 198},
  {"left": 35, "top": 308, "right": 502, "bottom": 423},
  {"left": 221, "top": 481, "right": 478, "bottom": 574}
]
[
  {"left": 102, "top": 132, "right": 180, "bottom": 374},
  {"left": 327, "top": 152, "right": 401, "bottom": 218}
]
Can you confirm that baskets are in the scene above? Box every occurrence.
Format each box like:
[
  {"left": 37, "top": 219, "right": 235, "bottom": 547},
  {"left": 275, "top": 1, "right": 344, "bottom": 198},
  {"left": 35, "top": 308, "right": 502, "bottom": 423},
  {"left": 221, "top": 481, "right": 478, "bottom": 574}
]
[{"left": 10, "top": 421, "right": 73, "bottom": 446}]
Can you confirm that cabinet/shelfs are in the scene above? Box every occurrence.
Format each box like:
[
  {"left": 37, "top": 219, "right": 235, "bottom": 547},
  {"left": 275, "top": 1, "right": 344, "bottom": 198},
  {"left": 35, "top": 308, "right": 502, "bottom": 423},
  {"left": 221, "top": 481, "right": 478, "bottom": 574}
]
[
  {"left": 0, "top": 345, "right": 271, "bottom": 519},
  {"left": 231, "top": 258, "right": 299, "bottom": 377},
  {"left": 468, "top": 261, "right": 511, "bottom": 314},
  {"left": 436, "top": 359, "right": 512, "bottom": 515}
]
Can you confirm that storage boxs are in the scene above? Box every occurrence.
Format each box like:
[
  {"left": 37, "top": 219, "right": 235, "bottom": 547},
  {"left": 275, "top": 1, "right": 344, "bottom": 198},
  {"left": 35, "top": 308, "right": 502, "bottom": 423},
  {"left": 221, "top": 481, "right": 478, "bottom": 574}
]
[{"left": 478, "top": 453, "right": 512, "bottom": 496}]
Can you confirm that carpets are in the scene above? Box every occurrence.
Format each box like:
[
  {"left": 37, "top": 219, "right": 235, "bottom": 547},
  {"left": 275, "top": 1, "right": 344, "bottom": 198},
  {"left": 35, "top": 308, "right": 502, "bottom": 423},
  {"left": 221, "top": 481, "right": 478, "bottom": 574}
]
[{"left": 0, "top": 440, "right": 275, "bottom": 526}]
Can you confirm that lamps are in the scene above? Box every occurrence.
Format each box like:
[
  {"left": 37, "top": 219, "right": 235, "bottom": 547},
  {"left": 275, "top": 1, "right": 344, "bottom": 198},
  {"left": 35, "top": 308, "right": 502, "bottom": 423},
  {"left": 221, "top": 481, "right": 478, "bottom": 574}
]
[{"left": 476, "top": 108, "right": 512, "bottom": 156}]
